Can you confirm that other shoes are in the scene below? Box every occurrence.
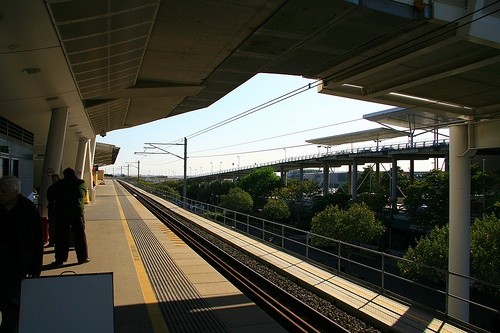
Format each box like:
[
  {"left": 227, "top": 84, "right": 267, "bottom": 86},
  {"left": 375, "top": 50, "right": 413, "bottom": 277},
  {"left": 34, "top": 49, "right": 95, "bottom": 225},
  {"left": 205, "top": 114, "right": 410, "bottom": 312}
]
[
  {"left": 78, "top": 258, "right": 90, "bottom": 264},
  {"left": 51, "top": 261, "right": 62, "bottom": 267}
]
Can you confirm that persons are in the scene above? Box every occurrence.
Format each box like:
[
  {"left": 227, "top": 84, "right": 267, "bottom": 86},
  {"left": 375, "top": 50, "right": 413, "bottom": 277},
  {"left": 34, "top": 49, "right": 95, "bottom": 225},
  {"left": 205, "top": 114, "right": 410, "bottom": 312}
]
[
  {"left": 0, "top": 176, "right": 44, "bottom": 333},
  {"left": 47, "top": 168, "right": 91, "bottom": 268}
]
